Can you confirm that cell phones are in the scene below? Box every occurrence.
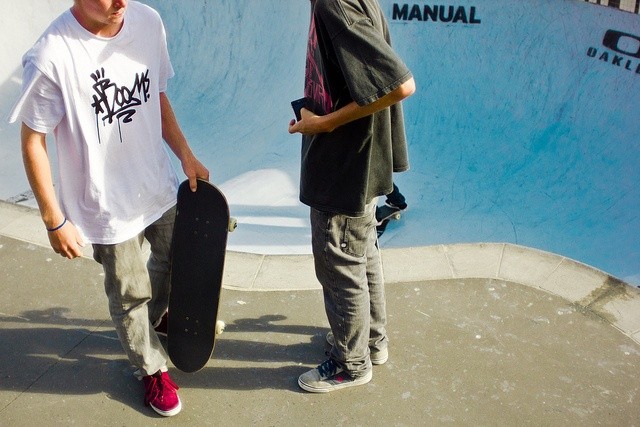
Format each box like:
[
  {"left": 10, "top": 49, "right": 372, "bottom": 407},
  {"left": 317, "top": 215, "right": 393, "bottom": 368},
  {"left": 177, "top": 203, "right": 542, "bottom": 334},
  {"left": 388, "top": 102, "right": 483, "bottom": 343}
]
[{"left": 290, "top": 96, "right": 319, "bottom": 121}]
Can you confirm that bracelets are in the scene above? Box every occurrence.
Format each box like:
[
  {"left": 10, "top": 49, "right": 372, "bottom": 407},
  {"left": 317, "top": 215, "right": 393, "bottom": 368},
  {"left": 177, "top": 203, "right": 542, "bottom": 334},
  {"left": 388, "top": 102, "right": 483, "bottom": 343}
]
[{"left": 46, "top": 217, "right": 68, "bottom": 231}]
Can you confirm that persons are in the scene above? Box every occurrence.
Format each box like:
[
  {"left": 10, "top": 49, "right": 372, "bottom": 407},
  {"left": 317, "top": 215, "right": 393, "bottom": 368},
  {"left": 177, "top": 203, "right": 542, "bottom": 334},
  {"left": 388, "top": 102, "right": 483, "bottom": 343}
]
[
  {"left": 287, "top": 0, "right": 417, "bottom": 394},
  {"left": 5, "top": 0, "right": 211, "bottom": 417},
  {"left": 375, "top": 181, "right": 409, "bottom": 227}
]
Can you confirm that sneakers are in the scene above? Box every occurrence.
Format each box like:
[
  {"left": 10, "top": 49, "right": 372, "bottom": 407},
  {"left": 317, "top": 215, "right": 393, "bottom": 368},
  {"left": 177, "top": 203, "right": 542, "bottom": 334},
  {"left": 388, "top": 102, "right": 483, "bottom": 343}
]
[
  {"left": 154, "top": 312, "right": 169, "bottom": 338},
  {"left": 326, "top": 328, "right": 388, "bottom": 365},
  {"left": 143, "top": 369, "right": 182, "bottom": 417},
  {"left": 298, "top": 352, "right": 372, "bottom": 393}
]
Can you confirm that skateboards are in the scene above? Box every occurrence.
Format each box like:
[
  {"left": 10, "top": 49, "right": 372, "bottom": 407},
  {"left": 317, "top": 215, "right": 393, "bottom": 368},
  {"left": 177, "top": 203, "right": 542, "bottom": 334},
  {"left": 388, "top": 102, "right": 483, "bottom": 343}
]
[
  {"left": 373, "top": 202, "right": 407, "bottom": 222},
  {"left": 167, "top": 178, "right": 237, "bottom": 373}
]
